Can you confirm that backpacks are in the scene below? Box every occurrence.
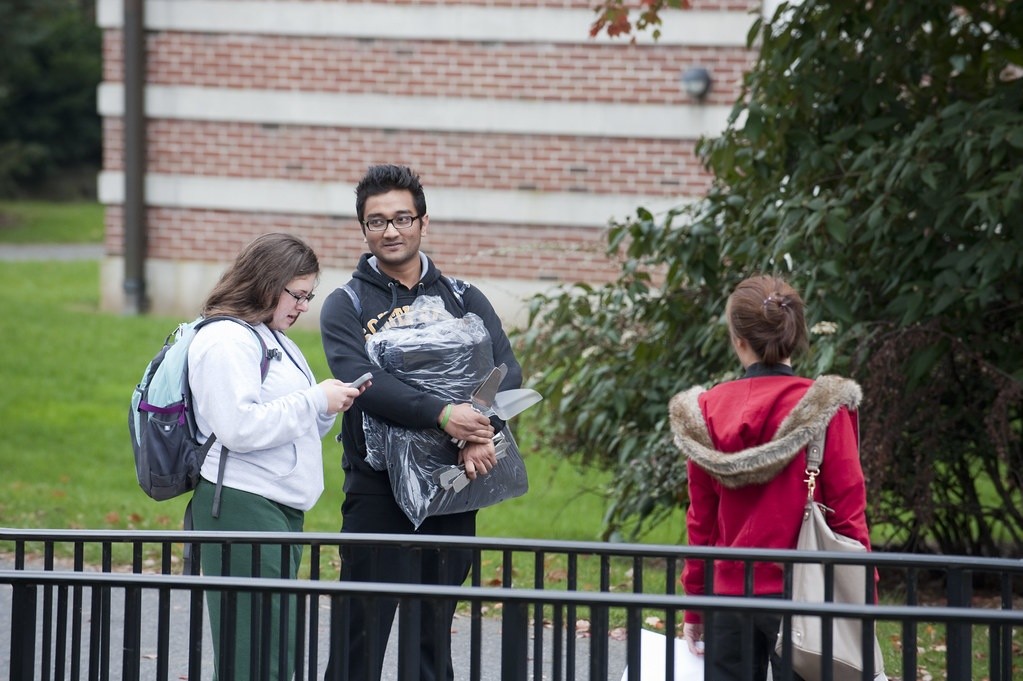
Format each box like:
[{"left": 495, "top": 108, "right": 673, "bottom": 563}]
[{"left": 127, "top": 314, "right": 271, "bottom": 501}]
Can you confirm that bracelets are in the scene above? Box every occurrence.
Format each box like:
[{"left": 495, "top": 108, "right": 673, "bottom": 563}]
[{"left": 439, "top": 402, "right": 452, "bottom": 429}]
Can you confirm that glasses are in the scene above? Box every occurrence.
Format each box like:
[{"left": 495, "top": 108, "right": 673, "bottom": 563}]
[
  {"left": 285, "top": 288, "right": 314, "bottom": 302},
  {"left": 362, "top": 215, "right": 419, "bottom": 231}
]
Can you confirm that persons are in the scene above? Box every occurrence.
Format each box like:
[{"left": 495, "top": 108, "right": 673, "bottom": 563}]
[
  {"left": 319, "top": 162, "right": 526, "bottom": 681},
  {"left": 666, "top": 272, "right": 878, "bottom": 681},
  {"left": 189, "top": 231, "right": 375, "bottom": 680}
]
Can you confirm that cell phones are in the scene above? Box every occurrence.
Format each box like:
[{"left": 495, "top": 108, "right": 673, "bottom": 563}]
[{"left": 348, "top": 372, "right": 373, "bottom": 389}]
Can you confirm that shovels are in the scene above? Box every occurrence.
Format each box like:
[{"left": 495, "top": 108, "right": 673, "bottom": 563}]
[
  {"left": 431, "top": 430, "right": 511, "bottom": 493},
  {"left": 480, "top": 388, "right": 544, "bottom": 421},
  {"left": 457, "top": 367, "right": 503, "bottom": 449},
  {"left": 451, "top": 362, "right": 508, "bottom": 444}
]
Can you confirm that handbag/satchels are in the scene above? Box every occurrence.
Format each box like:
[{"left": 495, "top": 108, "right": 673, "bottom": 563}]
[{"left": 774, "top": 425, "right": 885, "bottom": 681}]
